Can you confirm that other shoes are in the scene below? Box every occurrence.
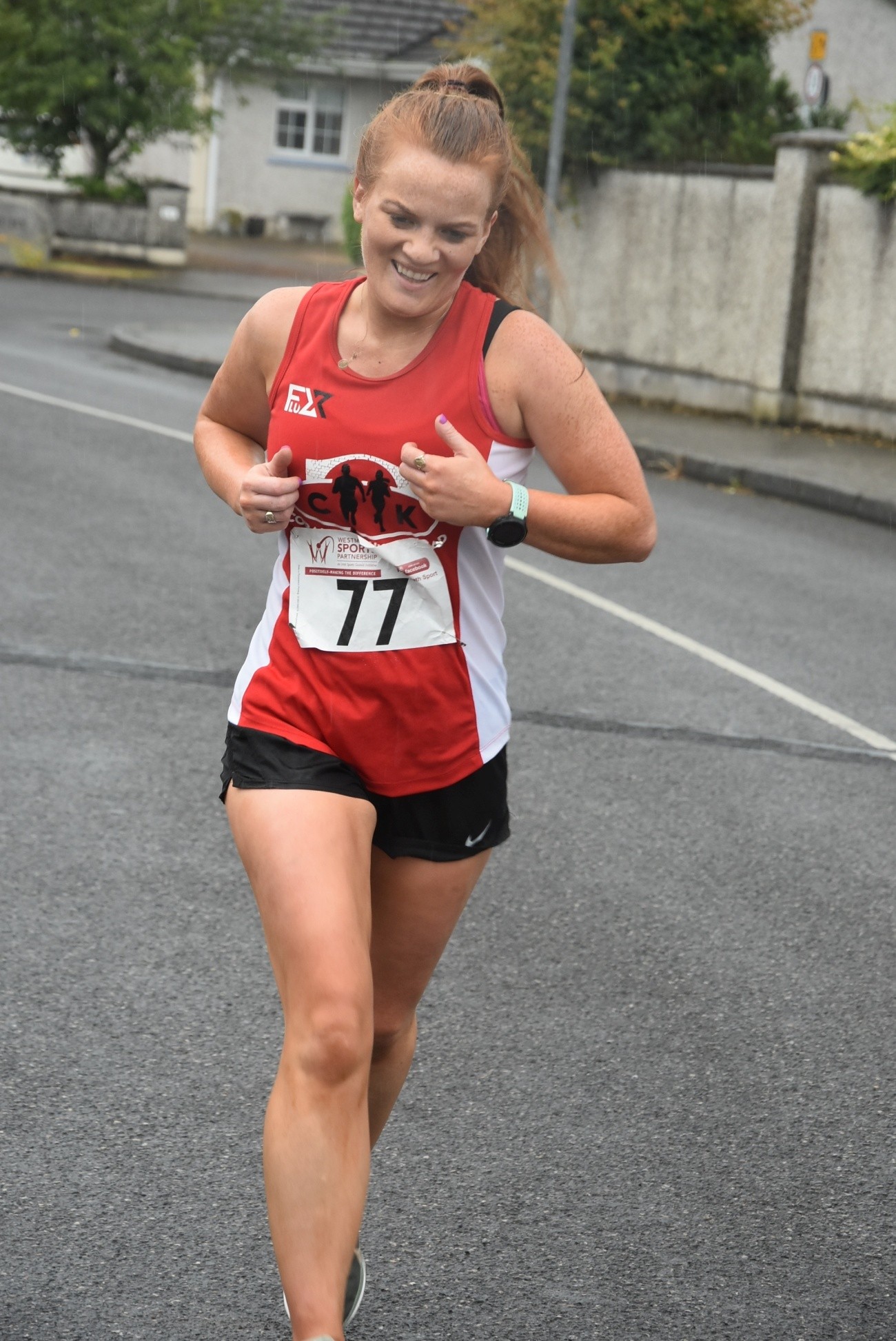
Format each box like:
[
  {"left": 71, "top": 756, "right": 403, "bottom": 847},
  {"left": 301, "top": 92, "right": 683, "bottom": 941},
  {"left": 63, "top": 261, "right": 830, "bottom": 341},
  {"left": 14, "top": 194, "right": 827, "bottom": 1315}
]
[{"left": 283, "top": 1233, "right": 366, "bottom": 1326}]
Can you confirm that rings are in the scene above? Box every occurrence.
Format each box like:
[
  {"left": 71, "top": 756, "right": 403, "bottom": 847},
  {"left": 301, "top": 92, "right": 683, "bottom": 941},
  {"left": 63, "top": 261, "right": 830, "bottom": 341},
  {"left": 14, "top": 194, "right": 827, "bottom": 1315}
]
[
  {"left": 265, "top": 511, "right": 277, "bottom": 524},
  {"left": 413, "top": 452, "right": 427, "bottom": 470}
]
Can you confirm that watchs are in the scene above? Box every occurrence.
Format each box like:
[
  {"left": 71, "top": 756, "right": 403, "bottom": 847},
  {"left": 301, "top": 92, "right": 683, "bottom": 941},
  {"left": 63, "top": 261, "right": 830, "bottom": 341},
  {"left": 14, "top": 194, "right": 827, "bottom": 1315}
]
[{"left": 485, "top": 477, "right": 529, "bottom": 549}]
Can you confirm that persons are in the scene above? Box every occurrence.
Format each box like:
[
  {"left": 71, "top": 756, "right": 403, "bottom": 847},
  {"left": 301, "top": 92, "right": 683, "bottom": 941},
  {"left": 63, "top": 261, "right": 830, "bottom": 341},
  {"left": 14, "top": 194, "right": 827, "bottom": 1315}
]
[{"left": 194, "top": 63, "right": 661, "bottom": 1341}]
